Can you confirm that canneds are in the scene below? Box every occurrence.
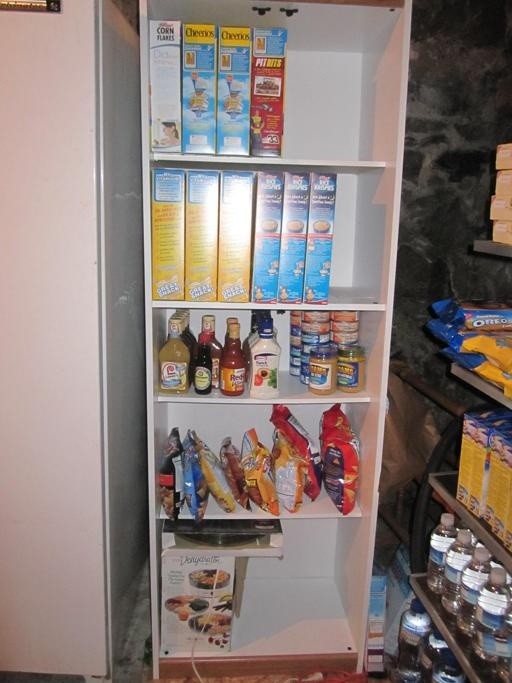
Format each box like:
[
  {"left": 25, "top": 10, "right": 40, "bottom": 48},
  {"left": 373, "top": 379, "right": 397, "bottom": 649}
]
[
  {"left": 290, "top": 311, "right": 368, "bottom": 349},
  {"left": 290, "top": 347, "right": 309, "bottom": 384},
  {"left": 309, "top": 347, "right": 338, "bottom": 395},
  {"left": 338, "top": 345, "right": 367, "bottom": 392}
]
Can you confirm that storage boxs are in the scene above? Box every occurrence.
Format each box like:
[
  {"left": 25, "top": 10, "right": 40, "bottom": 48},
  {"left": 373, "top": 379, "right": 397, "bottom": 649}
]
[{"left": 160, "top": 521, "right": 249, "bottom": 658}]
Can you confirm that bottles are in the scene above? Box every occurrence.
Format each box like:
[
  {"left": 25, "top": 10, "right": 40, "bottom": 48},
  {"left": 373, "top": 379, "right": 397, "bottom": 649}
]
[
  {"left": 220, "top": 323, "right": 245, "bottom": 396},
  {"left": 442, "top": 529, "right": 475, "bottom": 615},
  {"left": 433, "top": 648, "right": 467, "bottom": 683},
  {"left": 169, "top": 310, "right": 196, "bottom": 349},
  {"left": 457, "top": 548, "right": 491, "bottom": 631},
  {"left": 496, "top": 596, "right": 512, "bottom": 683},
  {"left": 224, "top": 318, "right": 238, "bottom": 349},
  {"left": 194, "top": 334, "right": 212, "bottom": 393},
  {"left": 251, "top": 318, "right": 281, "bottom": 399},
  {"left": 394, "top": 598, "right": 431, "bottom": 682},
  {"left": 472, "top": 568, "right": 512, "bottom": 663},
  {"left": 420, "top": 625, "right": 449, "bottom": 683},
  {"left": 427, "top": 514, "right": 457, "bottom": 592},
  {"left": 160, "top": 319, "right": 189, "bottom": 394},
  {"left": 247, "top": 313, "right": 267, "bottom": 348},
  {"left": 202, "top": 317, "right": 223, "bottom": 389}
]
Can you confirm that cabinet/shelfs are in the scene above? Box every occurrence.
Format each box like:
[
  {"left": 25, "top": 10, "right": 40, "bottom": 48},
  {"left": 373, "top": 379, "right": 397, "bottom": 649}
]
[
  {"left": 0, "top": 0, "right": 149, "bottom": 682},
  {"left": 384, "top": 239, "right": 512, "bottom": 682},
  {"left": 139, "top": 0, "right": 407, "bottom": 679}
]
